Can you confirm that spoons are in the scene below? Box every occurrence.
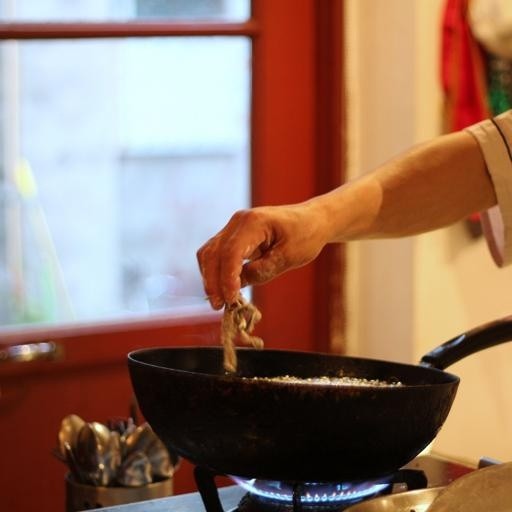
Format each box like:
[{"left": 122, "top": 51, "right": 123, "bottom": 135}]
[{"left": 58, "top": 411, "right": 181, "bottom": 488}]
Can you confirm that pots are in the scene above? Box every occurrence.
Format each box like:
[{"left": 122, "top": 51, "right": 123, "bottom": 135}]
[{"left": 126, "top": 317, "right": 512, "bottom": 486}]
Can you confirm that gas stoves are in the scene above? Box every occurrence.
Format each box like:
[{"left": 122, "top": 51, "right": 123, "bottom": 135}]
[{"left": 192, "top": 466, "right": 427, "bottom": 511}]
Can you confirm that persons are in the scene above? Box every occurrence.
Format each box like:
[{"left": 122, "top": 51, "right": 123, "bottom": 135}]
[{"left": 197, "top": 108, "right": 512, "bottom": 310}]
